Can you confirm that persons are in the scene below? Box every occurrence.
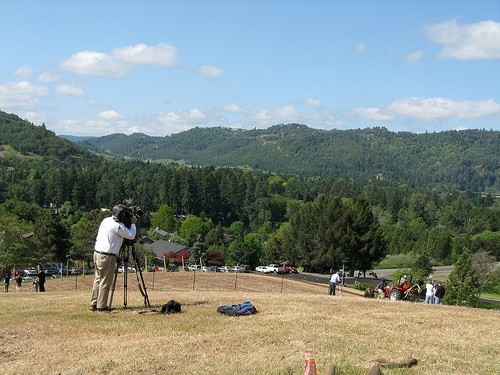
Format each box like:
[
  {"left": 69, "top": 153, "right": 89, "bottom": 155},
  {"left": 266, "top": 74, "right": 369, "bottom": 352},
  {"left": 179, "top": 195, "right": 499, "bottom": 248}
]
[
  {"left": 398, "top": 275, "right": 408, "bottom": 285},
  {"left": 37, "top": 268, "right": 46, "bottom": 292},
  {"left": 15, "top": 275, "right": 22, "bottom": 291},
  {"left": 377, "top": 280, "right": 385, "bottom": 300},
  {"left": 328, "top": 272, "right": 341, "bottom": 295},
  {"left": 426, "top": 279, "right": 446, "bottom": 305},
  {"left": 3, "top": 274, "right": 10, "bottom": 292},
  {"left": 89, "top": 204, "right": 137, "bottom": 311}
]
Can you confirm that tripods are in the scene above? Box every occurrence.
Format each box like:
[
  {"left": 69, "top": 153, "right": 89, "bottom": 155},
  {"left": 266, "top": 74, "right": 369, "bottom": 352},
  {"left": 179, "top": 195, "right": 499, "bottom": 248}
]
[{"left": 106, "top": 236, "right": 150, "bottom": 309}]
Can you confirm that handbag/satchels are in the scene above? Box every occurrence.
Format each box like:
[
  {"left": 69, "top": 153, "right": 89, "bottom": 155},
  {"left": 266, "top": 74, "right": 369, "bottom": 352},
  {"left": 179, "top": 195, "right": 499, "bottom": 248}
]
[{"left": 162, "top": 300, "right": 181, "bottom": 313}]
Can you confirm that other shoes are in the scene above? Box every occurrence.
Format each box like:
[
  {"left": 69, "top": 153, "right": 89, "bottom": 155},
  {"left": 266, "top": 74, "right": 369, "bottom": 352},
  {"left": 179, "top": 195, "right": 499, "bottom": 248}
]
[
  {"left": 97, "top": 307, "right": 116, "bottom": 312},
  {"left": 90, "top": 305, "right": 97, "bottom": 311}
]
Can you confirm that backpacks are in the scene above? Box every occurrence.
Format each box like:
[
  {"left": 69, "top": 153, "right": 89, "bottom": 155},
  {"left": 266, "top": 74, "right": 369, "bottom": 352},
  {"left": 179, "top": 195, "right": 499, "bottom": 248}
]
[{"left": 217, "top": 301, "right": 256, "bottom": 316}]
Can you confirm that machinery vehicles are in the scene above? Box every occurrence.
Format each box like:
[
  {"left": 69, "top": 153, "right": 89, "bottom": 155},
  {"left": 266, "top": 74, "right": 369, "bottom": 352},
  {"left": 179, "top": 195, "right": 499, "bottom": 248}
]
[{"left": 377, "top": 272, "right": 416, "bottom": 302}]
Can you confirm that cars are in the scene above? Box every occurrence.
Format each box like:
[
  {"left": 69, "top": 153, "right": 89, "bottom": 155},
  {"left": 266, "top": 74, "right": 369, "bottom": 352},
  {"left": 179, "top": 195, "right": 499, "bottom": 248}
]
[
  {"left": 255, "top": 266, "right": 271, "bottom": 274},
  {"left": 268, "top": 264, "right": 281, "bottom": 274},
  {"left": 117, "top": 264, "right": 143, "bottom": 274},
  {"left": 282, "top": 266, "right": 297, "bottom": 274},
  {"left": 187, "top": 264, "right": 250, "bottom": 274},
  {"left": 23, "top": 265, "right": 88, "bottom": 277}
]
